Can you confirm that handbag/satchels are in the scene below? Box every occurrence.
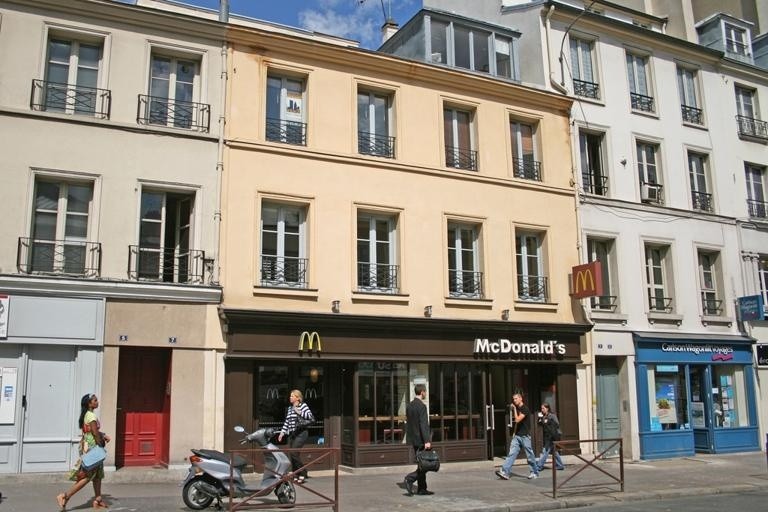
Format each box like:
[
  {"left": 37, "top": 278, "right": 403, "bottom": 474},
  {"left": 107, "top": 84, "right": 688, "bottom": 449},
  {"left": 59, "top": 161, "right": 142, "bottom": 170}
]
[
  {"left": 297, "top": 402, "right": 315, "bottom": 427},
  {"left": 551, "top": 427, "right": 562, "bottom": 441},
  {"left": 81, "top": 445, "right": 107, "bottom": 470},
  {"left": 416, "top": 450, "right": 440, "bottom": 472}
]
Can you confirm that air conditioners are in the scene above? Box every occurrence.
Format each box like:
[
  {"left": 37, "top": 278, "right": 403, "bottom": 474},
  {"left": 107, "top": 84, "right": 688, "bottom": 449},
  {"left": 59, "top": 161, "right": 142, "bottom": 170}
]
[{"left": 640, "top": 184, "right": 658, "bottom": 201}]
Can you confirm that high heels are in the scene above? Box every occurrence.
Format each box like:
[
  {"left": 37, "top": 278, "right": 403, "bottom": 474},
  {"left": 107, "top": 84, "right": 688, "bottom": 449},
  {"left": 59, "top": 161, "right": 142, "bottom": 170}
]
[
  {"left": 93, "top": 499, "right": 109, "bottom": 509},
  {"left": 56, "top": 492, "right": 70, "bottom": 511}
]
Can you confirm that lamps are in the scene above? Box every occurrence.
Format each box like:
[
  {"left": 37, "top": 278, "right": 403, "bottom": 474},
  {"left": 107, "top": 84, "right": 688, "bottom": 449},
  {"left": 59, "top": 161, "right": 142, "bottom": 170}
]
[
  {"left": 423, "top": 305, "right": 433, "bottom": 317},
  {"left": 500, "top": 308, "right": 510, "bottom": 320},
  {"left": 332, "top": 299, "right": 340, "bottom": 312}
]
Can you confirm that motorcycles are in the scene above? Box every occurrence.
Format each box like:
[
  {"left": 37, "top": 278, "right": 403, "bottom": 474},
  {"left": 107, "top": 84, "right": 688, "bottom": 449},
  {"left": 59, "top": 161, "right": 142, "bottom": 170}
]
[{"left": 180, "top": 426, "right": 296, "bottom": 510}]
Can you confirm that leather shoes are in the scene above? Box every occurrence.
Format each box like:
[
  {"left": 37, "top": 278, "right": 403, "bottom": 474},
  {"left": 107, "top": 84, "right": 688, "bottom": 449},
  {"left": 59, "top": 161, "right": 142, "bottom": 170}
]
[
  {"left": 403, "top": 479, "right": 414, "bottom": 494},
  {"left": 417, "top": 488, "right": 435, "bottom": 495}
]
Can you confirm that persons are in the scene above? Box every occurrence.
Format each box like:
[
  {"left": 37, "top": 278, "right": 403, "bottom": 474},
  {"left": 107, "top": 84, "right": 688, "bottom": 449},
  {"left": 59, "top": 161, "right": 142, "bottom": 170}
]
[
  {"left": 403, "top": 383, "right": 434, "bottom": 495},
  {"left": 278, "top": 388, "right": 313, "bottom": 484},
  {"left": 55, "top": 393, "right": 112, "bottom": 510},
  {"left": 536, "top": 401, "right": 564, "bottom": 471},
  {"left": 494, "top": 392, "right": 538, "bottom": 481}
]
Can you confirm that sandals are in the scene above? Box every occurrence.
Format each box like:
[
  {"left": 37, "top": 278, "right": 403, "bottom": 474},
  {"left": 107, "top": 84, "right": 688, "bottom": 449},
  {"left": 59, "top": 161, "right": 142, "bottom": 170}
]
[{"left": 293, "top": 473, "right": 307, "bottom": 483}]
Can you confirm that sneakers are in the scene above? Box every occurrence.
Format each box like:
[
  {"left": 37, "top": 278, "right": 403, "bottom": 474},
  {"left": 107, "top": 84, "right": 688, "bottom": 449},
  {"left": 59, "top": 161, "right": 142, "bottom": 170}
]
[
  {"left": 528, "top": 472, "right": 539, "bottom": 479},
  {"left": 495, "top": 471, "right": 509, "bottom": 480}
]
